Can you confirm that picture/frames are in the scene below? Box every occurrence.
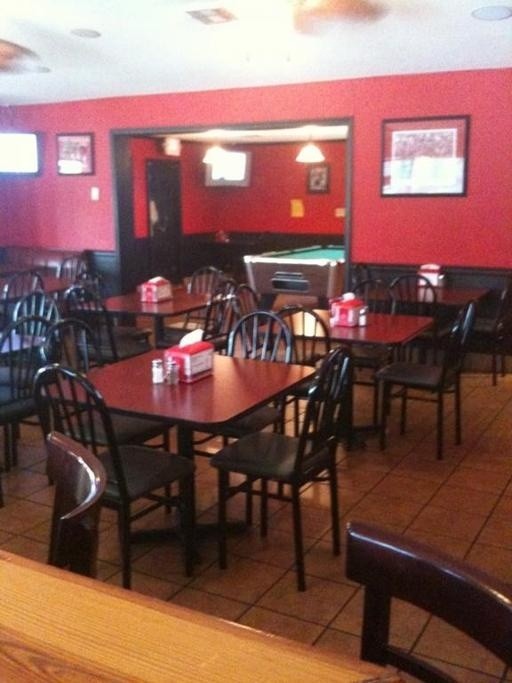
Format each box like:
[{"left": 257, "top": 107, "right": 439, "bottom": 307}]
[
  {"left": 305, "top": 163, "right": 330, "bottom": 193},
  {"left": 377, "top": 115, "right": 469, "bottom": 199},
  {"left": 56, "top": 131, "right": 94, "bottom": 178}
]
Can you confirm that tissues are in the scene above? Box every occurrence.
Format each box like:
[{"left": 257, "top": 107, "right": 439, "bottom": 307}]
[
  {"left": 163, "top": 328, "right": 215, "bottom": 382},
  {"left": 329, "top": 291, "right": 368, "bottom": 327},
  {"left": 417, "top": 263, "right": 446, "bottom": 287},
  {"left": 139, "top": 276, "right": 172, "bottom": 301}
]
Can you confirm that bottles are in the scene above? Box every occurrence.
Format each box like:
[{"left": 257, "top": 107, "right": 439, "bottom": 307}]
[
  {"left": 438, "top": 274, "right": 445, "bottom": 287},
  {"left": 358, "top": 309, "right": 368, "bottom": 326},
  {"left": 164, "top": 361, "right": 178, "bottom": 385},
  {"left": 152, "top": 358, "right": 164, "bottom": 384}
]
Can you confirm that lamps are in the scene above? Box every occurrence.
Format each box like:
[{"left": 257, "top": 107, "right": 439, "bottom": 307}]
[
  {"left": 294, "top": 140, "right": 326, "bottom": 165},
  {"left": 201, "top": 145, "right": 230, "bottom": 166}
]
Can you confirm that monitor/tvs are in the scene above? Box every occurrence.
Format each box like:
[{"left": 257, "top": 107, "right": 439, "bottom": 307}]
[{"left": 204, "top": 151, "right": 252, "bottom": 187}]
